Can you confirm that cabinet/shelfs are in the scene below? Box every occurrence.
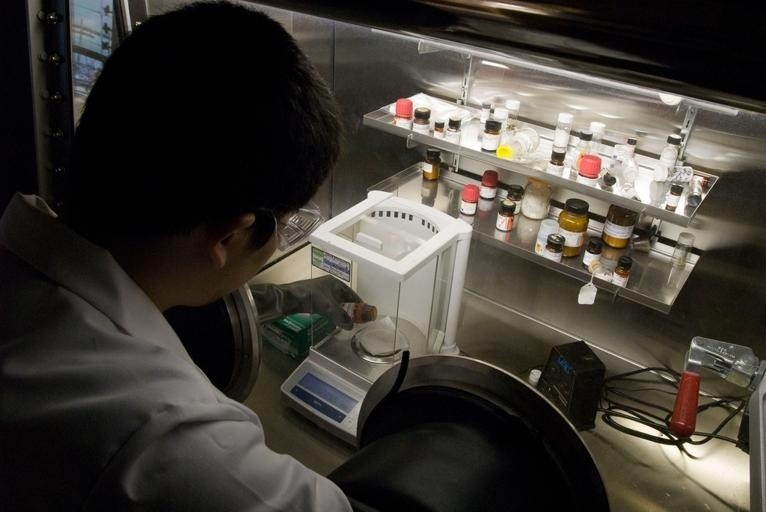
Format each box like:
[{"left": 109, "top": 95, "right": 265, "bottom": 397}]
[{"left": 359, "top": 92, "right": 720, "bottom": 315}]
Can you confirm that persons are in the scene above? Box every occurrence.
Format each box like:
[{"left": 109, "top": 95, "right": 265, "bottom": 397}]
[{"left": 0, "top": 0, "right": 363, "bottom": 512}]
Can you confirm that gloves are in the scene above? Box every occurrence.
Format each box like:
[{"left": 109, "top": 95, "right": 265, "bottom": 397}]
[{"left": 249, "top": 274, "right": 363, "bottom": 331}]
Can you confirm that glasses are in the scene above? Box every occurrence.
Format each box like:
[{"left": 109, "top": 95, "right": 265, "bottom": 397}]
[{"left": 258, "top": 199, "right": 325, "bottom": 252}]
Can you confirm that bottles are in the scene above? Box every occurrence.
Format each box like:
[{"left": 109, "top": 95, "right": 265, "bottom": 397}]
[
  {"left": 338, "top": 302, "right": 377, "bottom": 323},
  {"left": 395, "top": 96, "right": 707, "bottom": 287}
]
